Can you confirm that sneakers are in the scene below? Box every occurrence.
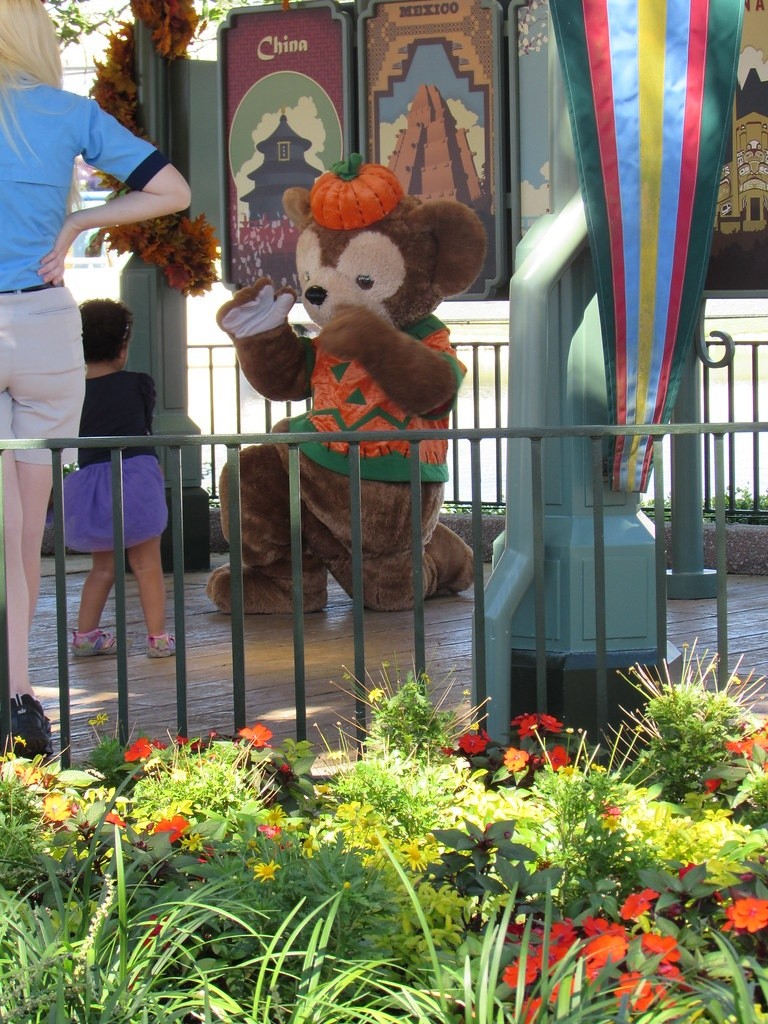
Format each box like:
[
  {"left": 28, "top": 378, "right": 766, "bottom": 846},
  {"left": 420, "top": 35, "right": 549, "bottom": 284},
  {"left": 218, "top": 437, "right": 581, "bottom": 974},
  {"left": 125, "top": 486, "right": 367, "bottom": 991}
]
[
  {"left": 70, "top": 629, "right": 132, "bottom": 657},
  {"left": 10, "top": 693, "right": 54, "bottom": 760},
  {"left": 145, "top": 635, "right": 177, "bottom": 658}
]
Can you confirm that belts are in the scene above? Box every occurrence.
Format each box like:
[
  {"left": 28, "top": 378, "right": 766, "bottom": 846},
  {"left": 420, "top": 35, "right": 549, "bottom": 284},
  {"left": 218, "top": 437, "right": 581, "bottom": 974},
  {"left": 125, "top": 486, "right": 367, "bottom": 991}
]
[{"left": 0, "top": 277, "right": 65, "bottom": 294}]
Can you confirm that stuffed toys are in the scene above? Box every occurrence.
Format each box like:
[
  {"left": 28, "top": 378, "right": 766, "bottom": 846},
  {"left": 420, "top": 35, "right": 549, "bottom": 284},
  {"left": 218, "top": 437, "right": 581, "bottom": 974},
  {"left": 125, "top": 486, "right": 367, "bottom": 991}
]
[{"left": 204, "top": 153, "right": 486, "bottom": 616}]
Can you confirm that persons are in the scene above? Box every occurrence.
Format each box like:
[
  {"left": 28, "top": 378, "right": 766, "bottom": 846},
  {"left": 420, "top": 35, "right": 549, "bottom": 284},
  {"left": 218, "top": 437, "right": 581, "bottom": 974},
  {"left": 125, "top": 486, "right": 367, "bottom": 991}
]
[
  {"left": 0, "top": 0, "right": 192, "bottom": 760},
  {"left": 62, "top": 299, "right": 175, "bottom": 658}
]
